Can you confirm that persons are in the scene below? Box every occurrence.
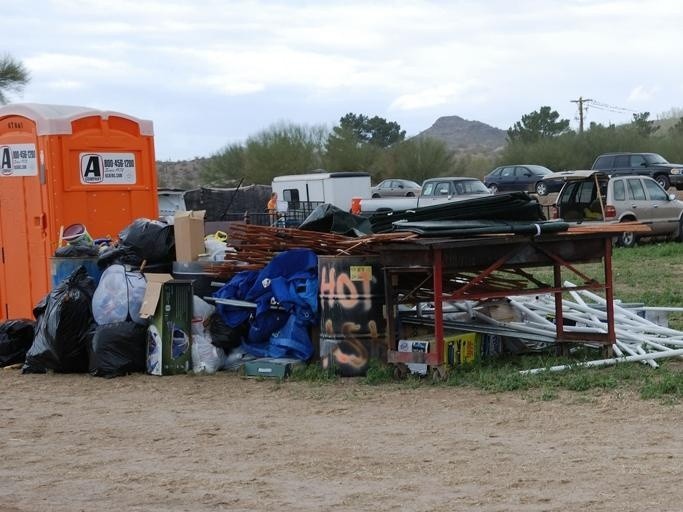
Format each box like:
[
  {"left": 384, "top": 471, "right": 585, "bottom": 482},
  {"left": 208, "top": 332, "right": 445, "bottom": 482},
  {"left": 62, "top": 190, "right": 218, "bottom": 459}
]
[{"left": 267, "top": 192, "right": 278, "bottom": 226}]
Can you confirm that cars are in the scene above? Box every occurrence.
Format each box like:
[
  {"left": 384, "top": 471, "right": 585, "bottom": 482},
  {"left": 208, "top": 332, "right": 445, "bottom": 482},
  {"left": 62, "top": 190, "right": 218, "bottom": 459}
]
[
  {"left": 371, "top": 179, "right": 422, "bottom": 197},
  {"left": 483, "top": 153, "right": 683, "bottom": 248}
]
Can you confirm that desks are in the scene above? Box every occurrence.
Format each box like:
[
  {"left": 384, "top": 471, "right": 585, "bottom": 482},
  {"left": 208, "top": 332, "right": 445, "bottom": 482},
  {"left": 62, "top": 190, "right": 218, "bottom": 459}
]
[{"left": 375, "top": 228, "right": 624, "bottom": 385}]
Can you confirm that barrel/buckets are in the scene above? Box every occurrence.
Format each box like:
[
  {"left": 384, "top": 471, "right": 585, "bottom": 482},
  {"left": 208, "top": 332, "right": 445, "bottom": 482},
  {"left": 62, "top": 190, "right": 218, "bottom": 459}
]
[
  {"left": 45, "top": 254, "right": 106, "bottom": 293},
  {"left": 61, "top": 224, "right": 94, "bottom": 246}
]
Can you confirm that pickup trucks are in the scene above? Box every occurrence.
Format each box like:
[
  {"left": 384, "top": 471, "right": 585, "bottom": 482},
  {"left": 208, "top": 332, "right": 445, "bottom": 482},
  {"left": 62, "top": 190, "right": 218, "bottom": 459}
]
[{"left": 352, "top": 176, "right": 493, "bottom": 216}]
